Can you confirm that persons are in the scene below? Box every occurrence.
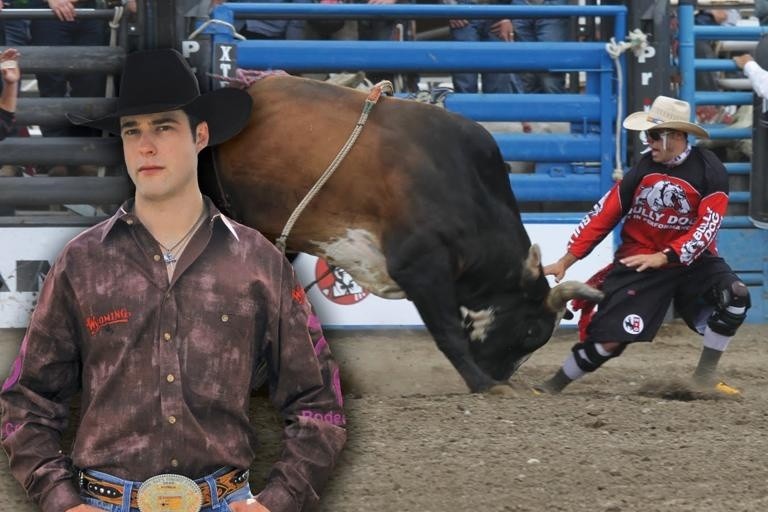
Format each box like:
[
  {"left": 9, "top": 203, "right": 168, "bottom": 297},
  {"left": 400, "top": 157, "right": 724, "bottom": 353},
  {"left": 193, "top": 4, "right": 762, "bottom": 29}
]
[
  {"left": 526, "top": 94, "right": 752, "bottom": 403},
  {"left": 0, "top": 1, "right": 768, "bottom": 231},
  {"left": 1, "top": 44, "right": 348, "bottom": 512}
]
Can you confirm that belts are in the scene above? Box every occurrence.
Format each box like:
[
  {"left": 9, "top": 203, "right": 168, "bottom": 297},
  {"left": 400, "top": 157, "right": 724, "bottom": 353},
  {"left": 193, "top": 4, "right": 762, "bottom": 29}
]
[{"left": 77, "top": 468, "right": 249, "bottom": 512}]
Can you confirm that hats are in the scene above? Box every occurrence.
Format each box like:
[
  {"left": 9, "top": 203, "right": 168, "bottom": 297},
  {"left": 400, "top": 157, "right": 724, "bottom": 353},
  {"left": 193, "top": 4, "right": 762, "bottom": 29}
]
[
  {"left": 66, "top": 48, "right": 252, "bottom": 147},
  {"left": 623, "top": 97, "right": 710, "bottom": 139}
]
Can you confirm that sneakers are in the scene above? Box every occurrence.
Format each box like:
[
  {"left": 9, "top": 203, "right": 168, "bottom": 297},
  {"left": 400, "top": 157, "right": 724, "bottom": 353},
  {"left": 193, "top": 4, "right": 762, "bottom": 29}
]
[
  {"left": 48, "top": 165, "right": 97, "bottom": 177},
  {"left": 0, "top": 164, "right": 23, "bottom": 177},
  {"left": 690, "top": 374, "right": 741, "bottom": 398},
  {"left": 532, "top": 381, "right": 556, "bottom": 397}
]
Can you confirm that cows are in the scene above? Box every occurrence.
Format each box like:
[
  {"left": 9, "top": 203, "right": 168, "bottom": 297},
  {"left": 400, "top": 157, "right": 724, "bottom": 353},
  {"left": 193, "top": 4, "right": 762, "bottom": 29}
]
[{"left": 197, "top": 75, "right": 606, "bottom": 396}]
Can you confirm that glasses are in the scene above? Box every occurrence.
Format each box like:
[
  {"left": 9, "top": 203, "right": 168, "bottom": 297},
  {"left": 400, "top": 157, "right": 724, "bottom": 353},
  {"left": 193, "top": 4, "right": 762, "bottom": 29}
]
[{"left": 644, "top": 130, "right": 675, "bottom": 140}]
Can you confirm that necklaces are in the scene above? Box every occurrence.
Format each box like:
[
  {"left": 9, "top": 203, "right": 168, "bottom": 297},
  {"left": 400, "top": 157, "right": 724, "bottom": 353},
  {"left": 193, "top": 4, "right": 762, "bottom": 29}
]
[{"left": 155, "top": 207, "right": 209, "bottom": 263}]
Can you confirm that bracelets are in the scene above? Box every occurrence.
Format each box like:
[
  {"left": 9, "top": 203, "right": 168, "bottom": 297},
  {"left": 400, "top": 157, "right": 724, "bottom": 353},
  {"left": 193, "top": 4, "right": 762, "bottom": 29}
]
[{"left": 662, "top": 246, "right": 679, "bottom": 262}]
